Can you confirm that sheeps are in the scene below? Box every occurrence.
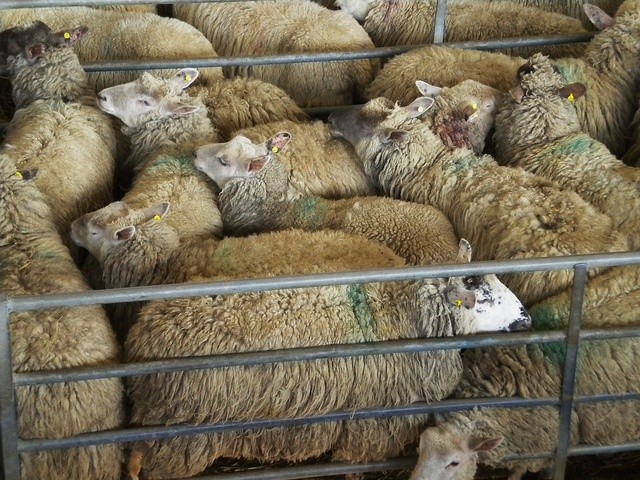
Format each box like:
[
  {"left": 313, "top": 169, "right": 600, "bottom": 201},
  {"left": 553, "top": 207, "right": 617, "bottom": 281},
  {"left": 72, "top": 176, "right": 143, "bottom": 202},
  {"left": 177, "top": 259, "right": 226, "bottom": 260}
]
[{"left": 0, "top": 0, "right": 640, "bottom": 479}]
